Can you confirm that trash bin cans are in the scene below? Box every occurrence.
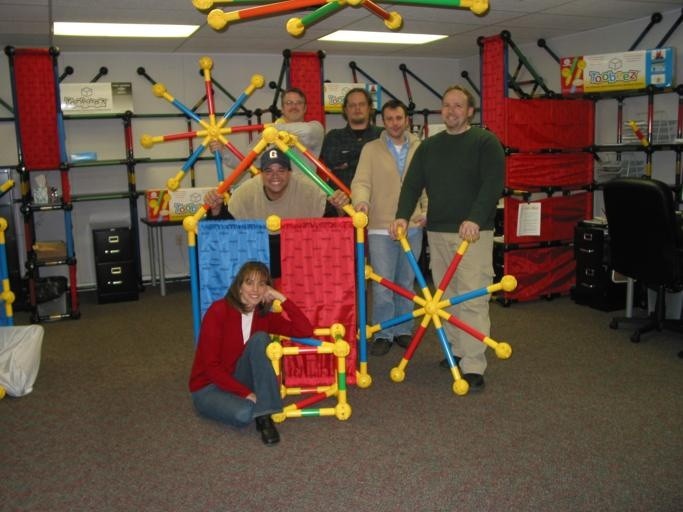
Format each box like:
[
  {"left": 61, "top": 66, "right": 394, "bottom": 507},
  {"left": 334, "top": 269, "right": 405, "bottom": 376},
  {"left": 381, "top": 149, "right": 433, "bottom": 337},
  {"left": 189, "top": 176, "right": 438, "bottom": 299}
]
[{"left": 35, "top": 276, "right": 67, "bottom": 316}]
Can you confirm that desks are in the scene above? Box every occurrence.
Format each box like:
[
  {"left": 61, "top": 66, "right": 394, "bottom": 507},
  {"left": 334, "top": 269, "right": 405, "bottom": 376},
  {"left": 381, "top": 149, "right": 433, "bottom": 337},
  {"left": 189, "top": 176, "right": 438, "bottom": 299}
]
[{"left": 140, "top": 218, "right": 183, "bottom": 297}]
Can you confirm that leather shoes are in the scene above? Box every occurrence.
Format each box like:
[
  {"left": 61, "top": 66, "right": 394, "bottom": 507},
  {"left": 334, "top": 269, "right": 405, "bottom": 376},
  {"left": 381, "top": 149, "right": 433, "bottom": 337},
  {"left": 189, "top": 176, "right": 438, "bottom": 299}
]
[
  {"left": 441, "top": 355, "right": 485, "bottom": 394},
  {"left": 370, "top": 335, "right": 413, "bottom": 357},
  {"left": 255, "top": 414, "right": 281, "bottom": 446}
]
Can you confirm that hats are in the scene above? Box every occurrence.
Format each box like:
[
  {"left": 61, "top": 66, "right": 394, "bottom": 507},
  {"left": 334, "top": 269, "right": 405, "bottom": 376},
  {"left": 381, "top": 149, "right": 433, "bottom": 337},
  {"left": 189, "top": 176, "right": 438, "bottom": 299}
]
[{"left": 261, "top": 148, "right": 291, "bottom": 171}]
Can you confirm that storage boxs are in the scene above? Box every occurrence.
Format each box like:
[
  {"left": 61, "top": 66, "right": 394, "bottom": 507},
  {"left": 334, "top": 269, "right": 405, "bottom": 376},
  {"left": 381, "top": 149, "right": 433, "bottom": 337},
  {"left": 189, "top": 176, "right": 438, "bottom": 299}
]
[
  {"left": 560, "top": 47, "right": 677, "bottom": 97},
  {"left": 145, "top": 187, "right": 222, "bottom": 222},
  {"left": 58, "top": 82, "right": 135, "bottom": 115},
  {"left": 323, "top": 82, "right": 382, "bottom": 114}
]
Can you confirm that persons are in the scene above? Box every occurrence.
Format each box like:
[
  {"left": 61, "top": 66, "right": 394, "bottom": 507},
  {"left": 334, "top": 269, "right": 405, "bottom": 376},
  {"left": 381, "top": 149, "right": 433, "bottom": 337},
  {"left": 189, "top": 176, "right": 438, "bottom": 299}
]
[
  {"left": 203, "top": 147, "right": 351, "bottom": 276},
  {"left": 318, "top": 86, "right": 386, "bottom": 217},
  {"left": 349, "top": 95, "right": 428, "bottom": 357},
  {"left": 386, "top": 85, "right": 509, "bottom": 391},
  {"left": 206, "top": 86, "right": 324, "bottom": 176},
  {"left": 186, "top": 259, "right": 315, "bottom": 448}
]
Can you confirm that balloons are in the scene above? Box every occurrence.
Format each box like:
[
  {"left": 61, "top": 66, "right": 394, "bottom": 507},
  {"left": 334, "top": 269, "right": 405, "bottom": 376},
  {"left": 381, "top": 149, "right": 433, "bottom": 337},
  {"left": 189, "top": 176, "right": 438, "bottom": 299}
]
[{"left": 182, "top": 119, "right": 373, "bottom": 390}]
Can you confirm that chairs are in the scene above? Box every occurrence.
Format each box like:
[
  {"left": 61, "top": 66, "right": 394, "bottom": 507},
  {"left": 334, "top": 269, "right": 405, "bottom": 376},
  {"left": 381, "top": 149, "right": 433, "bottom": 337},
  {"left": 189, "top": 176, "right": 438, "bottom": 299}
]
[{"left": 602, "top": 175, "right": 683, "bottom": 343}]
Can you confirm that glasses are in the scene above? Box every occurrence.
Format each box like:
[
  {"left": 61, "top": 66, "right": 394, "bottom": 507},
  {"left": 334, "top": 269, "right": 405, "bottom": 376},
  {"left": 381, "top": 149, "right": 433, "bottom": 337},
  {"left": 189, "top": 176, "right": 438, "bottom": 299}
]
[{"left": 285, "top": 100, "right": 304, "bottom": 105}]
